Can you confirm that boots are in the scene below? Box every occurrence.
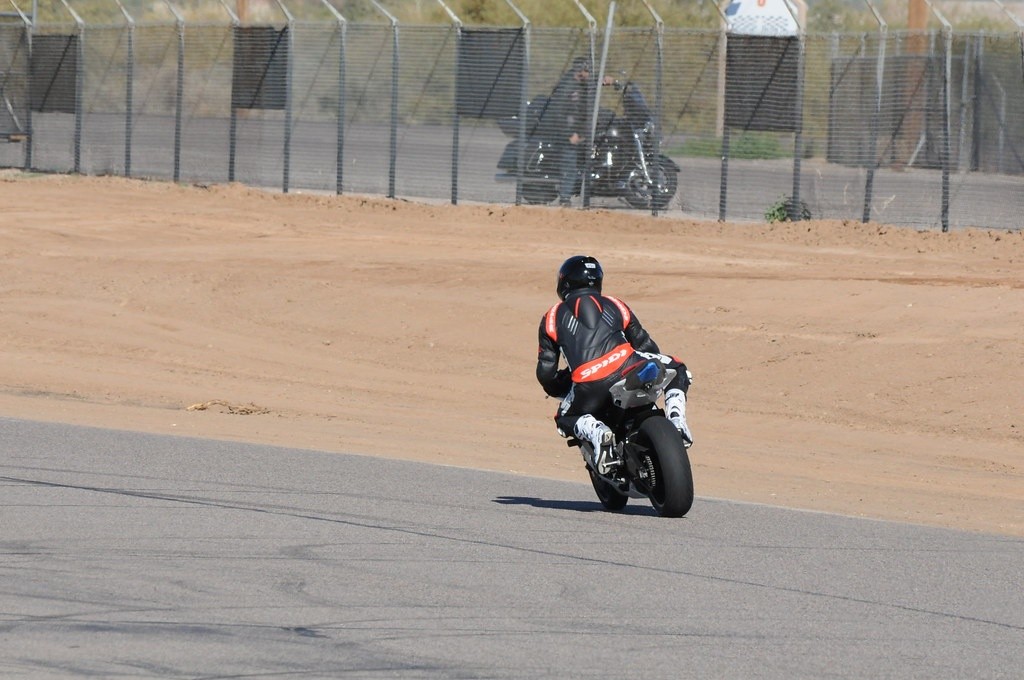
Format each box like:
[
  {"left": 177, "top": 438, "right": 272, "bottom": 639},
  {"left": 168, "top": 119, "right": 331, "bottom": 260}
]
[
  {"left": 573, "top": 414, "right": 616, "bottom": 474},
  {"left": 664, "top": 388, "right": 694, "bottom": 450}
]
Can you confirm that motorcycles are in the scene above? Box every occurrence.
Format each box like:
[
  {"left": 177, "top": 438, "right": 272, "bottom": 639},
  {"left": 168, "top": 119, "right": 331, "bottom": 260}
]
[
  {"left": 494, "top": 71, "right": 681, "bottom": 210},
  {"left": 545, "top": 357, "right": 695, "bottom": 518}
]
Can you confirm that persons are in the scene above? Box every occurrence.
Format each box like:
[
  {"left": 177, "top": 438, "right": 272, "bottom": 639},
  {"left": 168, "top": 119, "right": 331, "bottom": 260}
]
[
  {"left": 536, "top": 256, "right": 693, "bottom": 474},
  {"left": 549, "top": 55, "right": 615, "bottom": 209}
]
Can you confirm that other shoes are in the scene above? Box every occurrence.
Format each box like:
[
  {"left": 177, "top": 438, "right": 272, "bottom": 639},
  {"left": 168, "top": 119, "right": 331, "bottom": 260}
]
[{"left": 559, "top": 198, "right": 571, "bottom": 207}]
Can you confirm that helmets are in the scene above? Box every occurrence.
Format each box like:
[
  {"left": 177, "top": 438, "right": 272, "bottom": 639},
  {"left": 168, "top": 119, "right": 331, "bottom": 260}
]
[{"left": 556, "top": 256, "right": 605, "bottom": 298}]
